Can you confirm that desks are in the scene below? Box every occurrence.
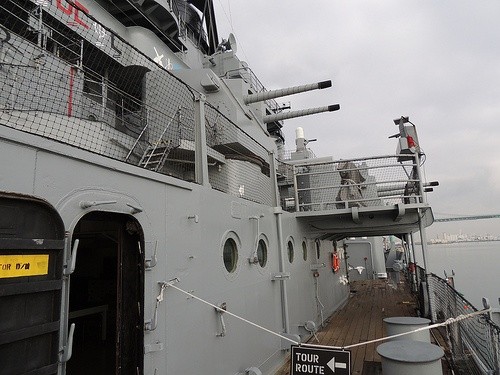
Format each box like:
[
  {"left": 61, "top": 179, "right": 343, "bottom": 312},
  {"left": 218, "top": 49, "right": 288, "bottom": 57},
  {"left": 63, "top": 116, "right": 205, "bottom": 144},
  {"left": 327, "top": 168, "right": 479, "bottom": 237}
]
[{"left": 69, "top": 304, "right": 109, "bottom": 343}]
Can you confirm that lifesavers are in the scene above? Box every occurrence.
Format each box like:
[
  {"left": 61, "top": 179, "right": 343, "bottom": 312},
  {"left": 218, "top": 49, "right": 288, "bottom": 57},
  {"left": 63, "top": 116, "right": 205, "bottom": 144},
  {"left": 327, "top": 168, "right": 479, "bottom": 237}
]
[{"left": 332, "top": 252, "right": 340, "bottom": 273}]
